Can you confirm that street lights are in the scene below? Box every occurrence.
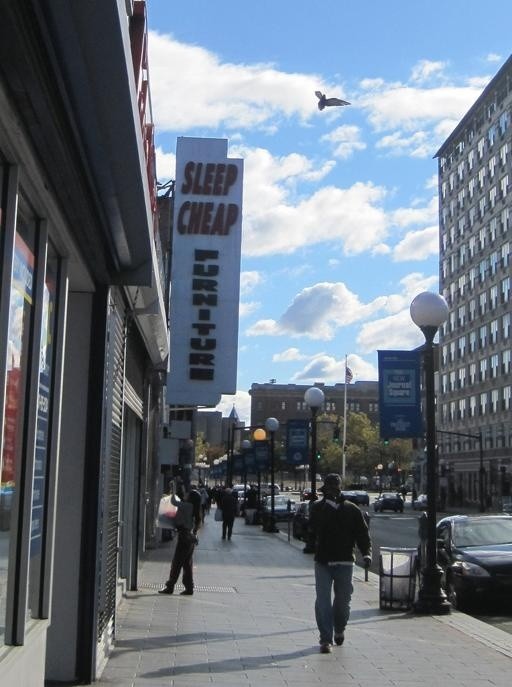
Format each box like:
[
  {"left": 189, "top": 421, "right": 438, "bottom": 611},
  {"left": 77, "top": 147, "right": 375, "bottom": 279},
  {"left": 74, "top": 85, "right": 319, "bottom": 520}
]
[
  {"left": 410, "top": 291, "right": 451, "bottom": 615},
  {"left": 205, "top": 417, "right": 280, "bottom": 533},
  {"left": 304, "top": 387, "right": 325, "bottom": 501}
]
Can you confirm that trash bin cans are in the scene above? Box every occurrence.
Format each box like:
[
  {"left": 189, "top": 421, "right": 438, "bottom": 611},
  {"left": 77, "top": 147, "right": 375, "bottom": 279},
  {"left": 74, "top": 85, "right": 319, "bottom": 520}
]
[
  {"left": 379, "top": 546, "right": 419, "bottom": 612},
  {"left": 245, "top": 508, "right": 257, "bottom": 525}
]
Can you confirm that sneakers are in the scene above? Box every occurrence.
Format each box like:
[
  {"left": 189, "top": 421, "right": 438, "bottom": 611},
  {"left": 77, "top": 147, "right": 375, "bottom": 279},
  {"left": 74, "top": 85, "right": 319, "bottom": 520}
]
[
  {"left": 157, "top": 586, "right": 194, "bottom": 596},
  {"left": 319, "top": 630, "right": 345, "bottom": 653}
]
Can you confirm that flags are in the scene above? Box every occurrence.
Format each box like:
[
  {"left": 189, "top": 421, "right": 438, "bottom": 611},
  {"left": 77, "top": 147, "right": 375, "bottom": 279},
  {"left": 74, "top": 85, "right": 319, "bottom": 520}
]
[{"left": 345, "top": 366, "right": 353, "bottom": 383}]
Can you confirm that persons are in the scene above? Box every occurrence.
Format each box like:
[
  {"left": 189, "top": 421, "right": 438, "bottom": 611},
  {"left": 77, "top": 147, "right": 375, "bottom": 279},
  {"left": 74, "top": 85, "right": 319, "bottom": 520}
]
[
  {"left": 159, "top": 480, "right": 201, "bottom": 594},
  {"left": 308, "top": 472, "right": 372, "bottom": 652},
  {"left": 186, "top": 482, "right": 257, "bottom": 540}
]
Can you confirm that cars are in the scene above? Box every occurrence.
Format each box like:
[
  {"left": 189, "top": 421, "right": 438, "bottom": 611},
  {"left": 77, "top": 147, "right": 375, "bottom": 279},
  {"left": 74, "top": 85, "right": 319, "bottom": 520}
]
[
  {"left": 260, "top": 495, "right": 296, "bottom": 523},
  {"left": 341, "top": 490, "right": 369, "bottom": 507},
  {"left": 300, "top": 488, "right": 319, "bottom": 502},
  {"left": 417, "top": 511, "right": 512, "bottom": 612},
  {"left": 292, "top": 500, "right": 370, "bottom": 540},
  {"left": 373, "top": 492, "right": 404, "bottom": 513}
]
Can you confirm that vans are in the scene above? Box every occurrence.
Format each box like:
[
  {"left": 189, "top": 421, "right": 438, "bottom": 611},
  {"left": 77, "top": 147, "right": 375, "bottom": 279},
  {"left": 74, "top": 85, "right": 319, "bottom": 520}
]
[{"left": 232, "top": 483, "right": 281, "bottom": 502}]
